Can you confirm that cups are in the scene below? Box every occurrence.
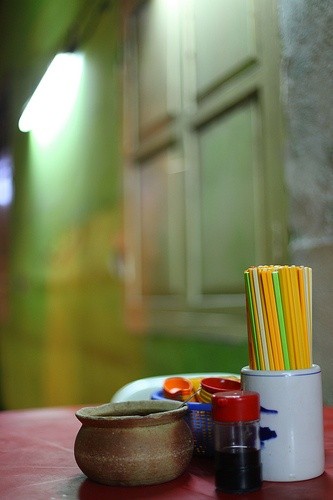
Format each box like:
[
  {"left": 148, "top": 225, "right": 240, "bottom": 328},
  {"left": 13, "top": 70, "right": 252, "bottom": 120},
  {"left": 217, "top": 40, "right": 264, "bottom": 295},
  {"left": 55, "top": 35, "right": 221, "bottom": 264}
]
[
  {"left": 213, "top": 390, "right": 262, "bottom": 494},
  {"left": 240, "top": 365, "right": 324, "bottom": 482}
]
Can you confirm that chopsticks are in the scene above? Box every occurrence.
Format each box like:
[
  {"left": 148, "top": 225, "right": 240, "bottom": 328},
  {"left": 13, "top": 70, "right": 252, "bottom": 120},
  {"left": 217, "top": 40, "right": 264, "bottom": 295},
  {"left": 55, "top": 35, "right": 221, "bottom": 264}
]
[{"left": 244, "top": 263, "right": 312, "bottom": 371}]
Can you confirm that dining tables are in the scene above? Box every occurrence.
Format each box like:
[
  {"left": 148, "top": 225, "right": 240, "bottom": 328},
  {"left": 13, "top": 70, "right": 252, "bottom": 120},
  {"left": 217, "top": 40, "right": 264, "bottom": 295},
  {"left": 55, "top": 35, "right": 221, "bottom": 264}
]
[{"left": 0, "top": 405, "right": 333, "bottom": 500}]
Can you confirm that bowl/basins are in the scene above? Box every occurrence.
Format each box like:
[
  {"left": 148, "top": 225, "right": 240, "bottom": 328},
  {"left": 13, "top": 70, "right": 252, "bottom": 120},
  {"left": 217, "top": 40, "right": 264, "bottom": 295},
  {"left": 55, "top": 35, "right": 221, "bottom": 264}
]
[
  {"left": 75, "top": 401, "right": 194, "bottom": 487},
  {"left": 200, "top": 377, "right": 241, "bottom": 403}
]
